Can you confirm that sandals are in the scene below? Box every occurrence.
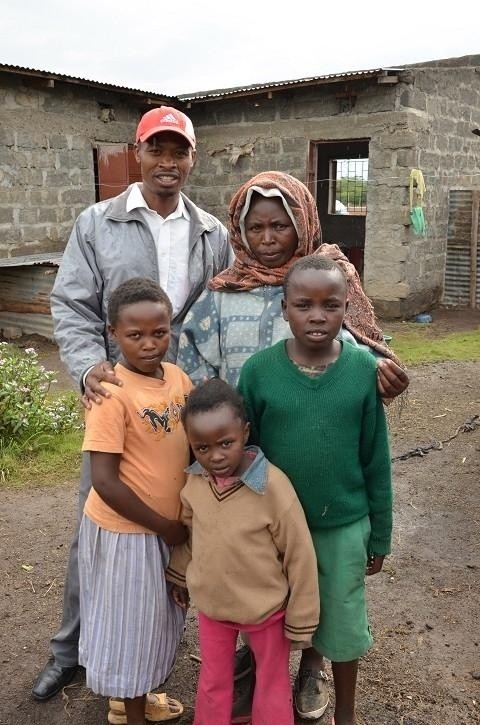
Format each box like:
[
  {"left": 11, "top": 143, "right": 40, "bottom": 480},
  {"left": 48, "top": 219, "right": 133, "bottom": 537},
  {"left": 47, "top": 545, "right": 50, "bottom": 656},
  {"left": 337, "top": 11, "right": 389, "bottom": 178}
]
[{"left": 107, "top": 692, "right": 183, "bottom": 724}]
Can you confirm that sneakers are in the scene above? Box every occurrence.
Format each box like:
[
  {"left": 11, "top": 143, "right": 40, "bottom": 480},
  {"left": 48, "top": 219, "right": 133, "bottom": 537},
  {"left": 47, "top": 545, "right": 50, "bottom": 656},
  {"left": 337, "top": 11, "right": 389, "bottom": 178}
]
[
  {"left": 295, "top": 656, "right": 330, "bottom": 721},
  {"left": 233, "top": 644, "right": 252, "bottom": 681}
]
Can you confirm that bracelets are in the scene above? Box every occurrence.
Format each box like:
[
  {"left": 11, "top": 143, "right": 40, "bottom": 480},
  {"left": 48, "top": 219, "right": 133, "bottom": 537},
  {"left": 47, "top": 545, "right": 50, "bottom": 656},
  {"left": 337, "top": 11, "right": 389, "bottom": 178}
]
[{"left": 82, "top": 365, "right": 96, "bottom": 387}]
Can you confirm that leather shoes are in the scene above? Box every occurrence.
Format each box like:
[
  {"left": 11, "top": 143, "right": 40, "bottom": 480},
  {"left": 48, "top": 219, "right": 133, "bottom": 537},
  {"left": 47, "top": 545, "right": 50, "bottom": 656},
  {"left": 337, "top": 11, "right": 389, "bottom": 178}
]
[{"left": 31, "top": 654, "right": 80, "bottom": 700}]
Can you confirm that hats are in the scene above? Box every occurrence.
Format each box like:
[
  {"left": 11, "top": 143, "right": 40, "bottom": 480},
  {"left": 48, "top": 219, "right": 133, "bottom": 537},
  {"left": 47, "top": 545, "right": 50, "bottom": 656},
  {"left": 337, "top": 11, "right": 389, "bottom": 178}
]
[{"left": 135, "top": 105, "right": 196, "bottom": 151}]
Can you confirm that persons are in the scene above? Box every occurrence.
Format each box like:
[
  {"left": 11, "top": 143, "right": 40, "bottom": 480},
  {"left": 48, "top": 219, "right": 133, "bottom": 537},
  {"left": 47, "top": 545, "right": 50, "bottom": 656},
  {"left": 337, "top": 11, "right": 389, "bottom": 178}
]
[
  {"left": 164, "top": 378, "right": 321, "bottom": 725},
  {"left": 235, "top": 248, "right": 398, "bottom": 725},
  {"left": 175, "top": 171, "right": 412, "bottom": 721},
  {"left": 77, "top": 279, "right": 198, "bottom": 725},
  {"left": 28, "top": 103, "right": 234, "bottom": 701}
]
